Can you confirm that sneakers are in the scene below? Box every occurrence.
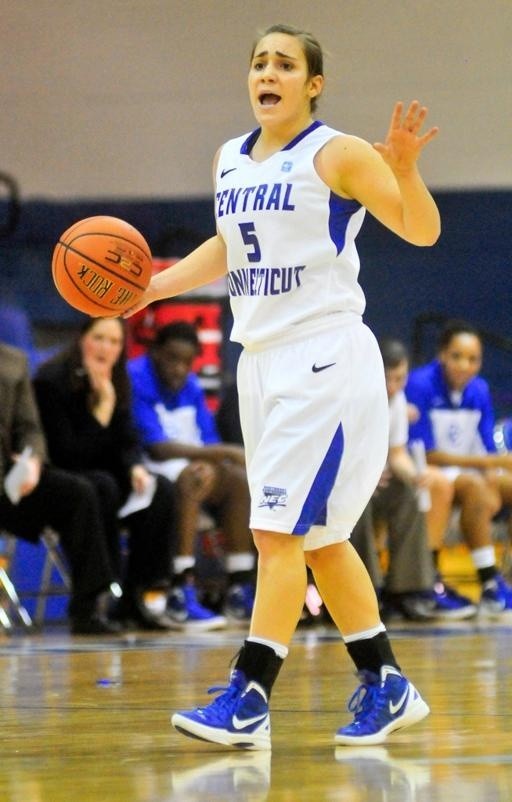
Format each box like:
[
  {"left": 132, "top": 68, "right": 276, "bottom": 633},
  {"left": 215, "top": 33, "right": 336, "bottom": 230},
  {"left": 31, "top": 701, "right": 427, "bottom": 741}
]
[
  {"left": 377, "top": 574, "right": 512, "bottom": 621},
  {"left": 168, "top": 669, "right": 271, "bottom": 750},
  {"left": 71, "top": 583, "right": 256, "bottom": 636},
  {"left": 335, "top": 664, "right": 431, "bottom": 745}
]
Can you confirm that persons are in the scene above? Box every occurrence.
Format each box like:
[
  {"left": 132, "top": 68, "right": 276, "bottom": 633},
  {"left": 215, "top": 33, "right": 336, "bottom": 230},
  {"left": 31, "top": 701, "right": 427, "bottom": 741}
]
[
  {"left": 348, "top": 338, "right": 442, "bottom": 622},
  {"left": 122, "top": 24, "right": 442, "bottom": 750},
  {"left": 1, "top": 342, "right": 121, "bottom": 634},
  {"left": 405, "top": 321, "right": 511, "bottom": 620},
  {"left": 125, "top": 321, "right": 257, "bottom": 631},
  {"left": 30, "top": 317, "right": 179, "bottom": 634}
]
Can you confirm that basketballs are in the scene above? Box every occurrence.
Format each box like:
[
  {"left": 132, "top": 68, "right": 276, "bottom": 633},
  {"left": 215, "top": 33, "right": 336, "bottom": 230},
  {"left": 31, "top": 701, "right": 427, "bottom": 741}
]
[{"left": 51, "top": 215, "right": 153, "bottom": 318}]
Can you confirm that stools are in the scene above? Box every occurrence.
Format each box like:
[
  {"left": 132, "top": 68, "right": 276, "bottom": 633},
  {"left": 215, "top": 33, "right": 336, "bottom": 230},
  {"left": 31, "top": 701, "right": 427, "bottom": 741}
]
[{"left": 0, "top": 529, "right": 74, "bottom": 632}]
